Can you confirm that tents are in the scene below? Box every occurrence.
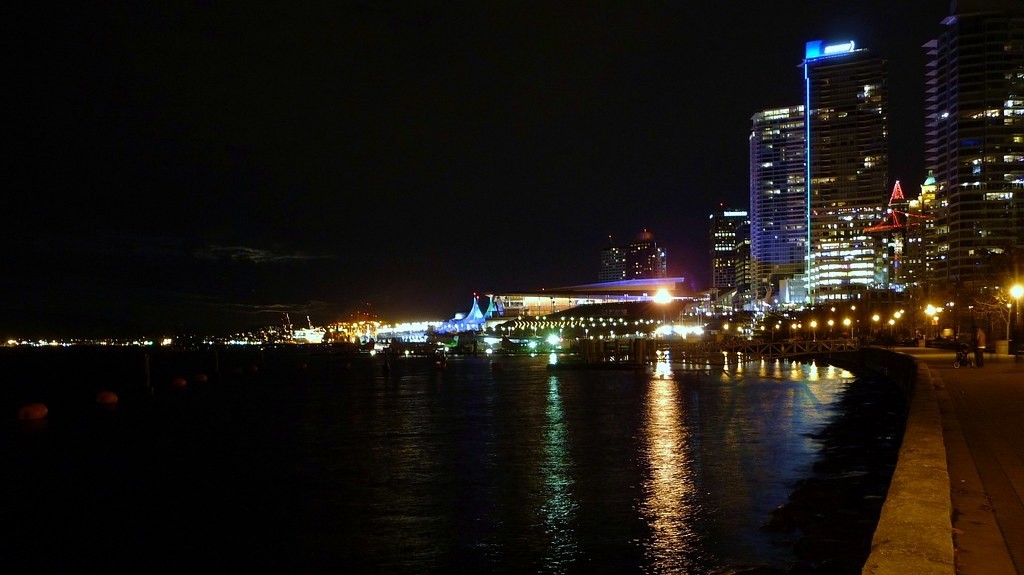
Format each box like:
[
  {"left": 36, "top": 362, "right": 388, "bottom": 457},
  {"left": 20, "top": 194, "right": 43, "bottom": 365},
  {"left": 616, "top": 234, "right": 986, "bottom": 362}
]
[{"left": 436, "top": 293, "right": 495, "bottom": 354}]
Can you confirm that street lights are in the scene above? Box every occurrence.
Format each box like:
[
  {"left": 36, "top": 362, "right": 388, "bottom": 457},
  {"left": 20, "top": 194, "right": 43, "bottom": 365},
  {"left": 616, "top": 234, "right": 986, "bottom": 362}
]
[{"left": 1008, "top": 282, "right": 1023, "bottom": 359}]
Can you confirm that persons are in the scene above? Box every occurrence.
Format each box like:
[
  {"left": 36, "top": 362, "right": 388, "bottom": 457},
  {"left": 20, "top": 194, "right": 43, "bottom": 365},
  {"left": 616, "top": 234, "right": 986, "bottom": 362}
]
[{"left": 973, "top": 326, "right": 987, "bottom": 368}]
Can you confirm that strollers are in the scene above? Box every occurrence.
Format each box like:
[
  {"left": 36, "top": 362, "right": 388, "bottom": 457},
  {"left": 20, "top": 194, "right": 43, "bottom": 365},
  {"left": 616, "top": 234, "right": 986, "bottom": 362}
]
[{"left": 952, "top": 344, "right": 975, "bottom": 368}]
